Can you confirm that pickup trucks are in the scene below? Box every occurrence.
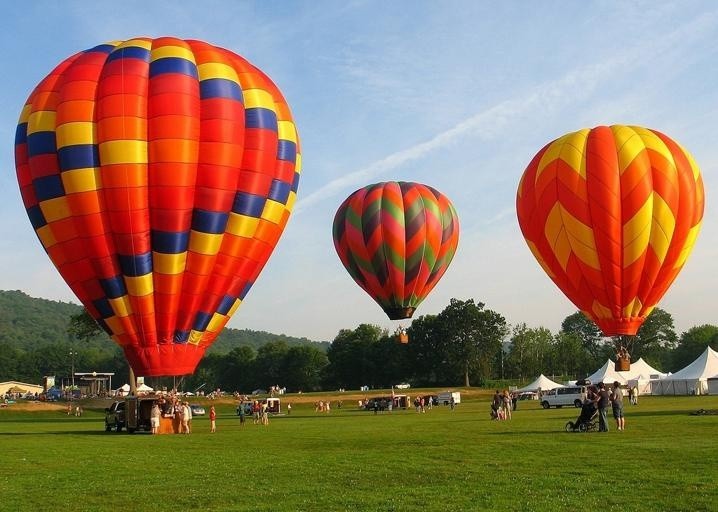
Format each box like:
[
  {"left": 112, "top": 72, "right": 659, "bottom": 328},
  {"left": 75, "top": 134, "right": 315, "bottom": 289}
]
[{"left": 105, "top": 401, "right": 125, "bottom": 432}]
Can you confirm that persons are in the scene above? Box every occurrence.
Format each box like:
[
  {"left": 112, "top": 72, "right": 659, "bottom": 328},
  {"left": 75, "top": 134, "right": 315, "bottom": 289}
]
[
  {"left": 288, "top": 403, "right": 291, "bottom": 414},
  {"left": 252, "top": 400, "right": 269, "bottom": 425},
  {"left": 315, "top": 399, "right": 331, "bottom": 413},
  {"left": 490, "top": 389, "right": 518, "bottom": 421},
  {"left": 210, "top": 407, "right": 216, "bottom": 432},
  {"left": 615, "top": 345, "right": 628, "bottom": 360},
  {"left": 364, "top": 397, "right": 393, "bottom": 415},
  {"left": 587, "top": 381, "right": 625, "bottom": 432},
  {"left": 239, "top": 400, "right": 245, "bottom": 427},
  {"left": 150, "top": 398, "right": 192, "bottom": 434},
  {"left": 414, "top": 395, "right": 433, "bottom": 413},
  {"left": 627, "top": 386, "right": 638, "bottom": 405},
  {"left": 450, "top": 397, "right": 454, "bottom": 409}
]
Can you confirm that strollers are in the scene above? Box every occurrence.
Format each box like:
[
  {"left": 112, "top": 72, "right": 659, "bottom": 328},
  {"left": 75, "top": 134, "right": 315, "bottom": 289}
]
[{"left": 566, "top": 399, "right": 599, "bottom": 431}]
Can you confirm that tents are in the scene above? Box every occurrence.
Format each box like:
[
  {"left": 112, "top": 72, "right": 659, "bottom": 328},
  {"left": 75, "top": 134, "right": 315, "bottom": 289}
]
[{"left": 591, "top": 346, "right": 718, "bottom": 394}]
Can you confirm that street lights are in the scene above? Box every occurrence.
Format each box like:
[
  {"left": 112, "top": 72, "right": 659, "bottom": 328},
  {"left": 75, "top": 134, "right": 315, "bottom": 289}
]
[{"left": 69, "top": 348, "right": 78, "bottom": 388}]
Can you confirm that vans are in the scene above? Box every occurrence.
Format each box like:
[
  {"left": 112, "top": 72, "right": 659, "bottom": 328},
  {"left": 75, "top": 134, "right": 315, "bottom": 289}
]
[
  {"left": 436, "top": 392, "right": 460, "bottom": 405},
  {"left": 540, "top": 386, "right": 588, "bottom": 408}
]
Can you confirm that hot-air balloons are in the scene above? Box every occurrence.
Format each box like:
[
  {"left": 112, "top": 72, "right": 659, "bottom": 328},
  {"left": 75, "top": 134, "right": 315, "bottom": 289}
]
[
  {"left": 15, "top": 36, "right": 302, "bottom": 434},
  {"left": 516, "top": 125, "right": 704, "bottom": 371},
  {"left": 333, "top": 181, "right": 459, "bottom": 344}
]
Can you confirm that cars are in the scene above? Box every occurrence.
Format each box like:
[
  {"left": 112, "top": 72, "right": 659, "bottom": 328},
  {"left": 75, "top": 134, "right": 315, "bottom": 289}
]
[
  {"left": 395, "top": 383, "right": 410, "bottom": 390},
  {"left": 191, "top": 404, "right": 206, "bottom": 416},
  {"left": 237, "top": 401, "right": 253, "bottom": 416},
  {"left": 414, "top": 395, "right": 438, "bottom": 407}
]
